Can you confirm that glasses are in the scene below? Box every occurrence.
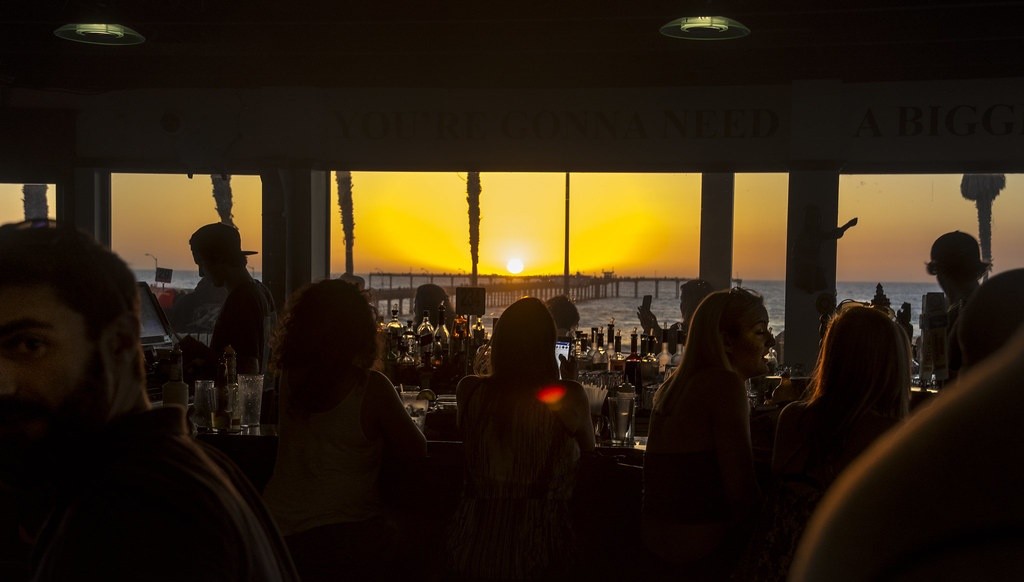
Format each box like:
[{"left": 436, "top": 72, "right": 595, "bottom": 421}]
[
  {"left": 836, "top": 298, "right": 900, "bottom": 325},
  {"left": 717, "top": 287, "right": 752, "bottom": 331}
]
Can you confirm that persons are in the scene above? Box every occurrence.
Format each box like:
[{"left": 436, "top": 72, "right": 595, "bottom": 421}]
[
  {"left": 547, "top": 296, "right": 580, "bottom": 338},
  {"left": 736, "top": 306, "right": 908, "bottom": 582},
  {"left": 174, "top": 223, "right": 277, "bottom": 425},
  {"left": 413, "top": 285, "right": 462, "bottom": 337},
  {"left": 638, "top": 289, "right": 776, "bottom": 582},
  {"left": 264, "top": 280, "right": 443, "bottom": 582},
  {"left": 0, "top": 219, "right": 303, "bottom": 582},
  {"left": 897, "top": 302, "right": 913, "bottom": 343},
  {"left": 447, "top": 297, "right": 595, "bottom": 582},
  {"left": 639, "top": 280, "right": 712, "bottom": 346},
  {"left": 930, "top": 231, "right": 997, "bottom": 390}
]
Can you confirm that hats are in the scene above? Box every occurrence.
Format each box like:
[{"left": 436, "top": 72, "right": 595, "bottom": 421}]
[
  {"left": 189, "top": 222, "right": 258, "bottom": 258},
  {"left": 931, "top": 230, "right": 990, "bottom": 272}
]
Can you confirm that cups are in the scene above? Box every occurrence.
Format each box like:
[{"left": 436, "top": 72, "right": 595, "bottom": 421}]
[
  {"left": 394, "top": 385, "right": 429, "bottom": 433},
  {"left": 433, "top": 395, "right": 457, "bottom": 433},
  {"left": 920, "top": 356, "right": 933, "bottom": 382},
  {"left": 237, "top": 374, "right": 264, "bottom": 431},
  {"left": 608, "top": 397, "right": 635, "bottom": 444},
  {"left": 195, "top": 359, "right": 239, "bottom": 432},
  {"left": 147, "top": 345, "right": 183, "bottom": 376},
  {"left": 590, "top": 414, "right": 608, "bottom": 446}
]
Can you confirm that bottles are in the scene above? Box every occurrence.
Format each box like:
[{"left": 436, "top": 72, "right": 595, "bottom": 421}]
[
  {"left": 377, "top": 316, "right": 387, "bottom": 360},
  {"left": 640, "top": 322, "right": 687, "bottom": 409},
  {"left": 435, "top": 315, "right": 466, "bottom": 367},
  {"left": 871, "top": 283, "right": 920, "bottom": 379},
  {"left": 417, "top": 310, "right": 435, "bottom": 367},
  {"left": 387, "top": 304, "right": 403, "bottom": 360},
  {"left": 573, "top": 328, "right": 608, "bottom": 370},
  {"left": 747, "top": 372, "right": 810, "bottom": 409},
  {"left": 608, "top": 324, "right": 642, "bottom": 410},
  {"left": 163, "top": 371, "right": 188, "bottom": 413},
  {"left": 766, "top": 359, "right": 815, "bottom": 377},
  {"left": 466, "top": 318, "right": 491, "bottom": 367},
  {"left": 617, "top": 375, "right": 635, "bottom": 410},
  {"left": 402, "top": 321, "right": 416, "bottom": 365}
]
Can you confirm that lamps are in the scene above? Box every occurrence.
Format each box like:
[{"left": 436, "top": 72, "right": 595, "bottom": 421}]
[
  {"left": 52, "top": 0, "right": 146, "bottom": 46},
  {"left": 658, "top": 0, "right": 752, "bottom": 41}
]
